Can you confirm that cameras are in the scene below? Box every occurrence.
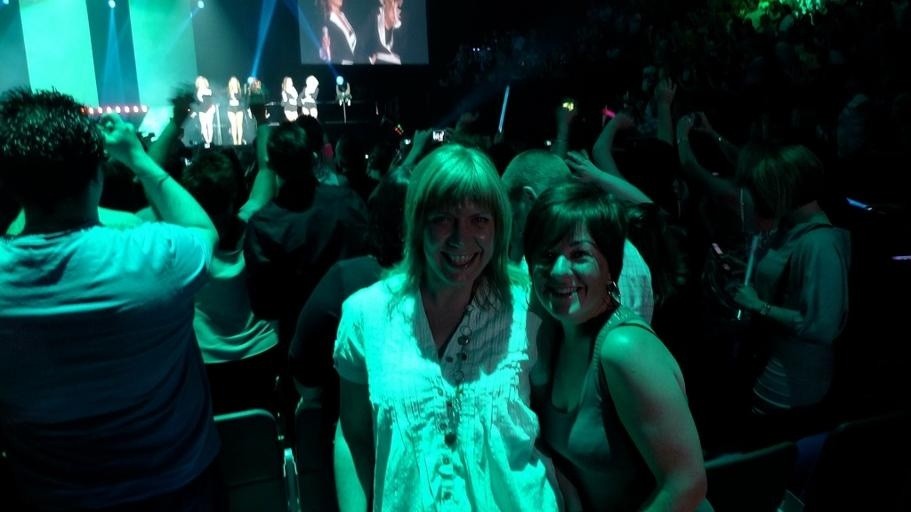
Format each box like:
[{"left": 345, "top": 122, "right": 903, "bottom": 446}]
[{"left": 431, "top": 129, "right": 446, "bottom": 143}]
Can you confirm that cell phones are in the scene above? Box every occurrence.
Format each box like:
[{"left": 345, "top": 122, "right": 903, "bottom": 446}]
[{"left": 710, "top": 242, "right": 725, "bottom": 260}]
